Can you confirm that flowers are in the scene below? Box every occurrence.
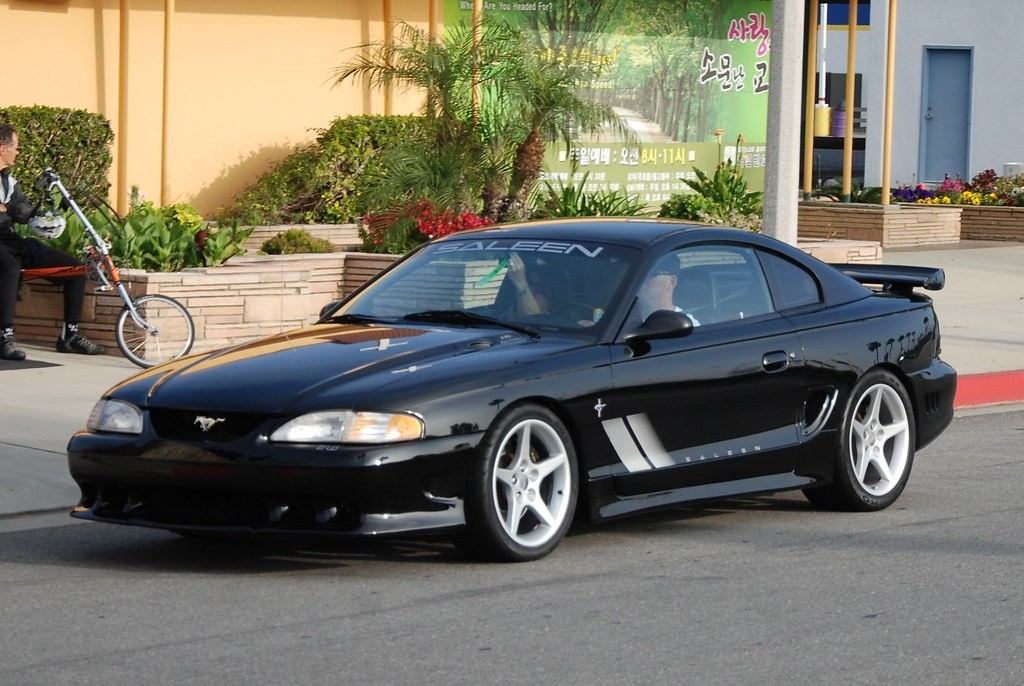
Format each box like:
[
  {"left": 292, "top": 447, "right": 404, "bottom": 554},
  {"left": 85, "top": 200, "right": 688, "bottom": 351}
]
[
  {"left": 889, "top": 169, "right": 1024, "bottom": 207},
  {"left": 677, "top": 129, "right": 764, "bottom": 221}
]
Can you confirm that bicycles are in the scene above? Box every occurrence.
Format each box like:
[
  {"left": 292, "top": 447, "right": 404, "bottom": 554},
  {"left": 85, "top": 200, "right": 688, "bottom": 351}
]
[{"left": 17, "top": 165, "right": 195, "bottom": 370}]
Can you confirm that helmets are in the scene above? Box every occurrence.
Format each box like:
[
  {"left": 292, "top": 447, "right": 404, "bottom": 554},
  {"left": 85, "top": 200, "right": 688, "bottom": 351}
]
[{"left": 29, "top": 214, "right": 67, "bottom": 240}]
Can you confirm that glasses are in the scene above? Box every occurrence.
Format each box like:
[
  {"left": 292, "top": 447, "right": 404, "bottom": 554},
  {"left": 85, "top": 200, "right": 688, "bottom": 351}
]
[
  {"left": 2, "top": 144, "right": 22, "bottom": 152},
  {"left": 651, "top": 271, "right": 674, "bottom": 277}
]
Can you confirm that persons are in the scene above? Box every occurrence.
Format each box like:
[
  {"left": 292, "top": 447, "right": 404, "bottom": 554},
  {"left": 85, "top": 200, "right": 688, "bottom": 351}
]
[
  {"left": 637, "top": 254, "right": 701, "bottom": 327},
  {"left": 0, "top": 124, "right": 105, "bottom": 361},
  {"left": 498, "top": 249, "right": 548, "bottom": 321}
]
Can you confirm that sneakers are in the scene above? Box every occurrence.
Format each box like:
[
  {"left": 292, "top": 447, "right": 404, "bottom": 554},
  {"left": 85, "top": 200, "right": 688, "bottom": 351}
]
[
  {"left": 0, "top": 334, "right": 26, "bottom": 361},
  {"left": 55, "top": 335, "right": 105, "bottom": 354}
]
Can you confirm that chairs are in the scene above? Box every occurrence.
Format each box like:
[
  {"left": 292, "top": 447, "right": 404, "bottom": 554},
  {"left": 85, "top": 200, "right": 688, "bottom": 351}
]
[{"left": 678, "top": 268, "right": 721, "bottom": 324}]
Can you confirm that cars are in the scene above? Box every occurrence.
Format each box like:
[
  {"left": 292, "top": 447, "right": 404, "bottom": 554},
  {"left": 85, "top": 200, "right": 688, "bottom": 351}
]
[{"left": 66, "top": 215, "right": 959, "bottom": 565}]
